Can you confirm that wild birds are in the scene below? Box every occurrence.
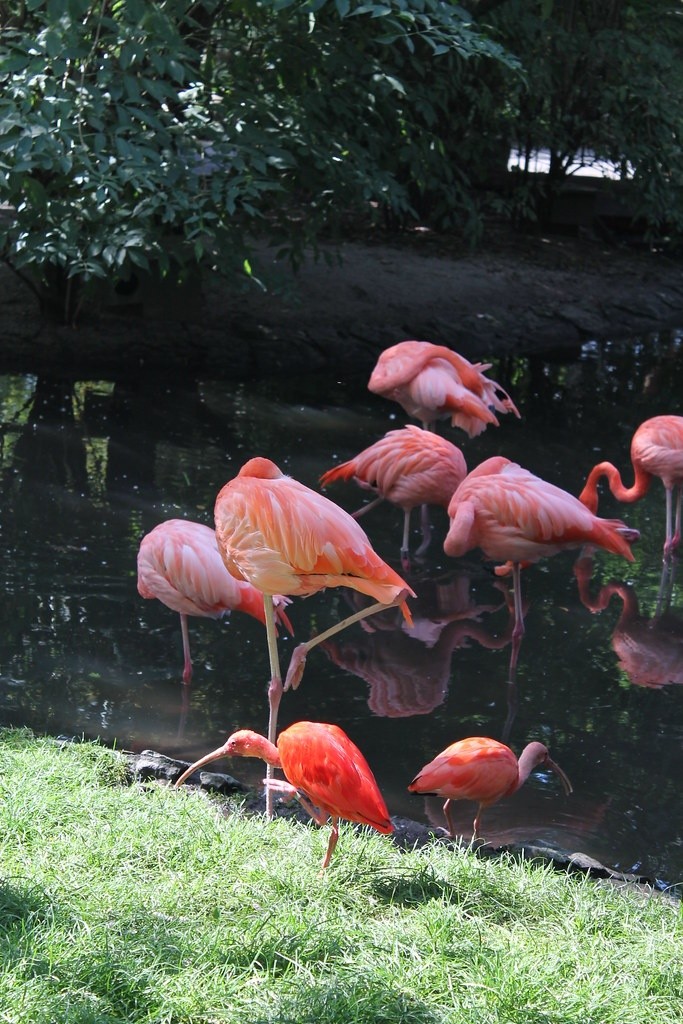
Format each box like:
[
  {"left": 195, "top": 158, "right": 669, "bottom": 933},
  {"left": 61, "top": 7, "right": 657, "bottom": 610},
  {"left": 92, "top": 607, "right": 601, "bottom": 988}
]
[
  {"left": 134, "top": 519, "right": 297, "bottom": 685},
  {"left": 173, "top": 720, "right": 395, "bottom": 870},
  {"left": 213, "top": 456, "right": 418, "bottom": 826},
  {"left": 441, "top": 454, "right": 638, "bottom": 643},
  {"left": 576, "top": 413, "right": 683, "bottom": 560},
  {"left": 408, "top": 736, "right": 575, "bottom": 843},
  {"left": 575, "top": 549, "right": 682, "bottom": 688},
  {"left": 367, "top": 338, "right": 521, "bottom": 537},
  {"left": 317, "top": 422, "right": 468, "bottom": 570},
  {"left": 304, "top": 504, "right": 531, "bottom": 719}
]
